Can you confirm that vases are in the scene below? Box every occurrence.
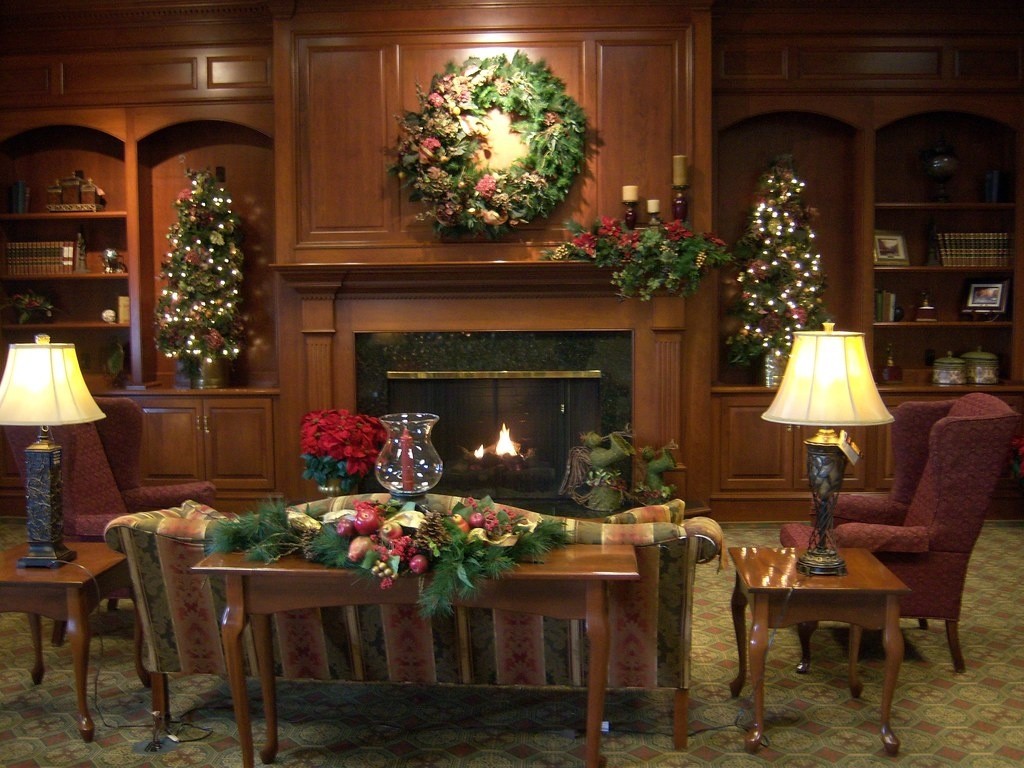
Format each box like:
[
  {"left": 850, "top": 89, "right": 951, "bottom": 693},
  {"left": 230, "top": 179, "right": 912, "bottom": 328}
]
[{"left": 317, "top": 479, "right": 344, "bottom": 498}]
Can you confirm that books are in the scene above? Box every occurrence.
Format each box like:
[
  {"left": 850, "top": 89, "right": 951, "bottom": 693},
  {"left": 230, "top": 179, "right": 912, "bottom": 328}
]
[
  {"left": 971, "top": 232, "right": 1012, "bottom": 268},
  {"left": 5, "top": 241, "right": 57, "bottom": 277},
  {"left": 55, "top": 241, "right": 76, "bottom": 274},
  {"left": 937, "top": 232, "right": 972, "bottom": 267}
]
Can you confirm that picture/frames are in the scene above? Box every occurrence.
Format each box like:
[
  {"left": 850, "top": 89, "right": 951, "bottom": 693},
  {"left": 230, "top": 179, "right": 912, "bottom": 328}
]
[
  {"left": 873, "top": 229, "right": 910, "bottom": 266},
  {"left": 960, "top": 277, "right": 1011, "bottom": 314}
]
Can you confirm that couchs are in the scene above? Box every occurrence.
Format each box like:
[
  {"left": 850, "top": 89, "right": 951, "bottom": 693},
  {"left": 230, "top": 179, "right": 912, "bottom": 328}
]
[
  {"left": 103, "top": 498, "right": 725, "bottom": 751},
  {"left": 781, "top": 392, "right": 1021, "bottom": 673}
]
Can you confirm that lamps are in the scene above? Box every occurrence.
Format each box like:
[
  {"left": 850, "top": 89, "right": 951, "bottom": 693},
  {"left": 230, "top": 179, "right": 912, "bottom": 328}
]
[
  {"left": 760, "top": 321, "right": 895, "bottom": 576},
  {"left": 0, "top": 333, "right": 107, "bottom": 568}
]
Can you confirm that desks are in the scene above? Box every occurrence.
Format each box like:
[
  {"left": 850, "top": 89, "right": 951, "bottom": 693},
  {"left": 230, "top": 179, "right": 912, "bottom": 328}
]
[
  {"left": 0, "top": 542, "right": 151, "bottom": 741},
  {"left": 190, "top": 545, "right": 639, "bottom": 768},
  {"left": 728, "top": 546, "right": 900, "bottom": 754}
]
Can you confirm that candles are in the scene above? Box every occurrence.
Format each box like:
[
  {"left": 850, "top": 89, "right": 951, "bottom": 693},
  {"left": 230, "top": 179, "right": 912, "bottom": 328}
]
[
  {"left": 647, "top": 200, "right": 660, "bottom": 213},
  {"left": 400, "top": 424, "right": 415, "bottom": 492},
  {"left": 622, "top": 185, "right": 639, "bottom": 202},
  {"left": 673, "top": 155, "right": 688, "bottom": 186}
]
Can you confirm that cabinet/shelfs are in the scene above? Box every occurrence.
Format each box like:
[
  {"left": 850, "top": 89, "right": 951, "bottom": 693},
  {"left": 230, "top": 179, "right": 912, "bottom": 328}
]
[
  {"left": 713, "top": 34, "right": 1024, "bottom": 527},
  {"left": 1, "top": 45, "right": 285, "bottom": 511}
]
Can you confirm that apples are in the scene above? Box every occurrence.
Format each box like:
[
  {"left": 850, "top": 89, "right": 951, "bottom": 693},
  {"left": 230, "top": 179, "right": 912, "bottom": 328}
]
[{"left": 337, "top": 509, "right": 486, "bottom": 573}]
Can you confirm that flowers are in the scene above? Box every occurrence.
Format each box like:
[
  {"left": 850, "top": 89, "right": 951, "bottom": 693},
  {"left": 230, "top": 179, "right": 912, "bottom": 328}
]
[
  {"left": 386, "top": 48, "right": 588, "bottom": 243},
  {"left": 299, "top": 408, "right": 388, "bottom": 494},
  {"left": 539, "top": 214, "right": 736, "bottom": 301}
]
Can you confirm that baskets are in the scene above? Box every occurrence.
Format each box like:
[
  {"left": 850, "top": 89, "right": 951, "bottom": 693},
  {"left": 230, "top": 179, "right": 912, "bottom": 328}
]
[
  {"left": 938, "top": 233, "right": 1013, "bottom": 267},
  {"left": 6, "top": 241, "right": 74, "bottom": 275}
]
